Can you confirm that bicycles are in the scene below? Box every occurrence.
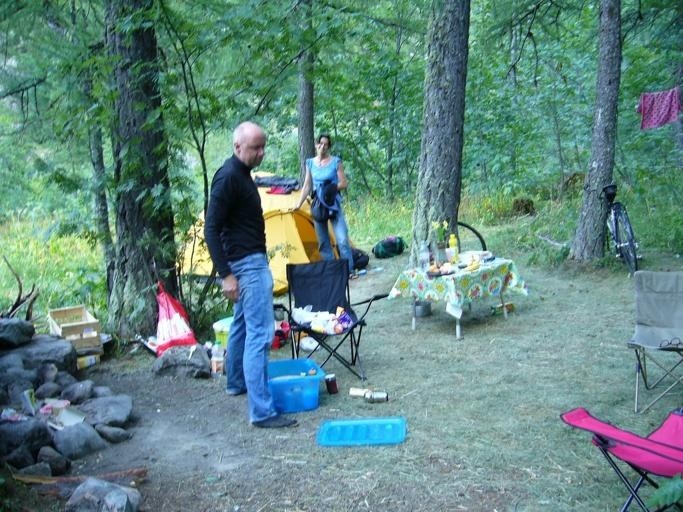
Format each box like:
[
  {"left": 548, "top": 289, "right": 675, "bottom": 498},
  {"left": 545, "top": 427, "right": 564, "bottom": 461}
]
[{"left": 600, "top": 178, "right": 642, "bottom": 276}]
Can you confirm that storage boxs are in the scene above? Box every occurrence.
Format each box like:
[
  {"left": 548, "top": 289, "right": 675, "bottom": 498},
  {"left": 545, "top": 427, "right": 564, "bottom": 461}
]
[
  {"left": 267, "top": 358, "right": 326, "bottom": 414},
  {"left": 212, "top": 316, "right": 233, "bottom": 348}
]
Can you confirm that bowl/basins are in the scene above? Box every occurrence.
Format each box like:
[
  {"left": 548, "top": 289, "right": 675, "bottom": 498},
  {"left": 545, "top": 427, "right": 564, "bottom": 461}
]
[{"left": 415, "top": 301, "right": 432, "bottom": 317}]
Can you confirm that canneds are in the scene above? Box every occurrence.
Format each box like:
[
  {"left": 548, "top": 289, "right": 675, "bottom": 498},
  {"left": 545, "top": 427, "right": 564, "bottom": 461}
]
[
  {"left": 365, "top": 393, "right": 388, "bottom": 403},
  {"left": 326, "top": 374, "right": 338, "bottom": 394}
]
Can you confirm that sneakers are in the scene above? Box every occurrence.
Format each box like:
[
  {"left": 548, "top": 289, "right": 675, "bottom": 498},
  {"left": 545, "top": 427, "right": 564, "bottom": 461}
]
[{"left": 250, "top": 414, "right": 299, "bottom": 430}]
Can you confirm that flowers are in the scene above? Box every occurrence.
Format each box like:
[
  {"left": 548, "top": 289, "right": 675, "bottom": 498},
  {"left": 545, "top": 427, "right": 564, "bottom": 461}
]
[{"left": 431, "top": 220, "right": 449, "bottom": 242}]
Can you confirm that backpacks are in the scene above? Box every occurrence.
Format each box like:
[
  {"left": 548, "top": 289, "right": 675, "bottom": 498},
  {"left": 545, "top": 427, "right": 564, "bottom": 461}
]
[{"left": 371, "top": 235, "right": 409, "bottom": 260}]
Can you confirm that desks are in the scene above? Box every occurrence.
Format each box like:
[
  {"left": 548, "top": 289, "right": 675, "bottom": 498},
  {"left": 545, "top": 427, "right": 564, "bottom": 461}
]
[{"left": 387, "top": 257, "right": 531, "bottom": 341}]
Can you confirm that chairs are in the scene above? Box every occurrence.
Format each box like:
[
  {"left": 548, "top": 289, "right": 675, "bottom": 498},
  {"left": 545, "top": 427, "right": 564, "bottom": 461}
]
[
  {"left": 560, "top": 406, "right": 683, "bottom": 512},
  {"left": 627, "top": 271, "right": 683, "bottom": 415},
  {"left": 274, "top": 258, "right": 390, "bottom": 382}
]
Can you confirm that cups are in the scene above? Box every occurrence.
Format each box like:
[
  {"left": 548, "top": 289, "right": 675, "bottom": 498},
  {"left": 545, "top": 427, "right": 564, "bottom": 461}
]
[{"left": 323, "top": 373, "right": 338, "bottom": 396}]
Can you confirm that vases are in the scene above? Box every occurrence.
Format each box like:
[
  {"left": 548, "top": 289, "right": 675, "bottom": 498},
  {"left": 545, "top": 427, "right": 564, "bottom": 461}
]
[{"left": 436, "top": 241, "right": 450, "bottom": 269}]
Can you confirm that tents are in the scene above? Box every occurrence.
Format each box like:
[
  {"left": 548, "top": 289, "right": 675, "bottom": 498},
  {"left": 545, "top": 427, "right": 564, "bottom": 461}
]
[{"left": 182, "top": 170, "right": 355, "bottom": 295}]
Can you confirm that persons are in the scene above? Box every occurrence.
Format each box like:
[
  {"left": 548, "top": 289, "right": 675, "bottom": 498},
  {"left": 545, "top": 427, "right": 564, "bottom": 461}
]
[
  {"left": 290, "top": 133, "right": 355, "bottom": 280},
  {"left": 204, "top": 120, "right": 299, "bottom": 428}
]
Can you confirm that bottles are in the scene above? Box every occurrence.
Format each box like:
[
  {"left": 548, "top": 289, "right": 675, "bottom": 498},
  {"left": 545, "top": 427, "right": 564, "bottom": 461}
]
[
  {"left": 348, "top": 386, "right": 389, "bottom": 403},
  {"left": 210, "top": 340, "right": 225, "bottom": 381},
  {"left": 409, "top": 233, "right": 459, "bottom": 270}
]
